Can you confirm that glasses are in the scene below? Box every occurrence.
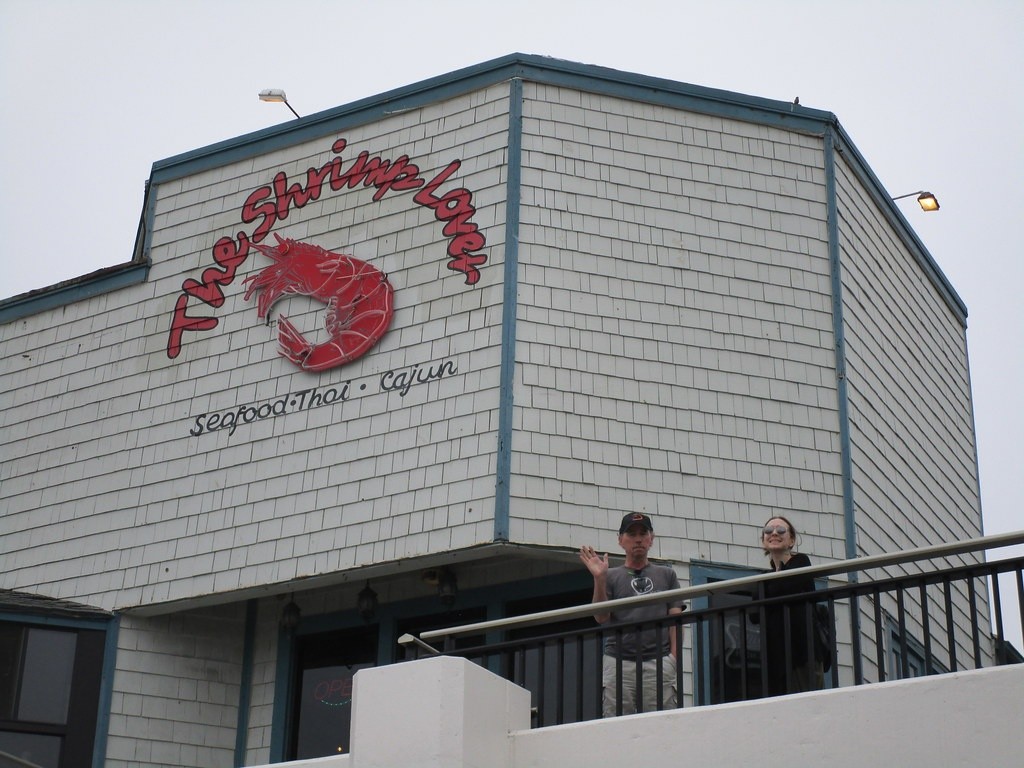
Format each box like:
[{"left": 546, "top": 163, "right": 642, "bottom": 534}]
[{"left": 764, "top": 526, "right": 789, "bottom": 534}]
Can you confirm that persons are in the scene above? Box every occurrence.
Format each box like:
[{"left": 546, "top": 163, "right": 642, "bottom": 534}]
[
  {"left": 579, "top": 511, "right": 687, "bottom": 721},
  {"left": 749, "top": 516, "right": 824, "bottom": 697}
]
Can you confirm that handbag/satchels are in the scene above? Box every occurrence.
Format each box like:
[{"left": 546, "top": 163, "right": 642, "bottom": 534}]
[{"left": 815, "top": 603, "right": 836, "bottom": 673}]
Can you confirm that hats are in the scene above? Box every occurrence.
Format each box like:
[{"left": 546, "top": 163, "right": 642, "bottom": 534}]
[{"left": 620, "top": 512, "right": 653, "bottom": 532}]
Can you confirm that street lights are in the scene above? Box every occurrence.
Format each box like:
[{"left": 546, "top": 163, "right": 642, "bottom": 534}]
[
  {"left": 893, "top": 190, "right": 940, "bottom": 212},
  {"left": 258, "top": 88, "right": 301, "bottom": 119}
]
